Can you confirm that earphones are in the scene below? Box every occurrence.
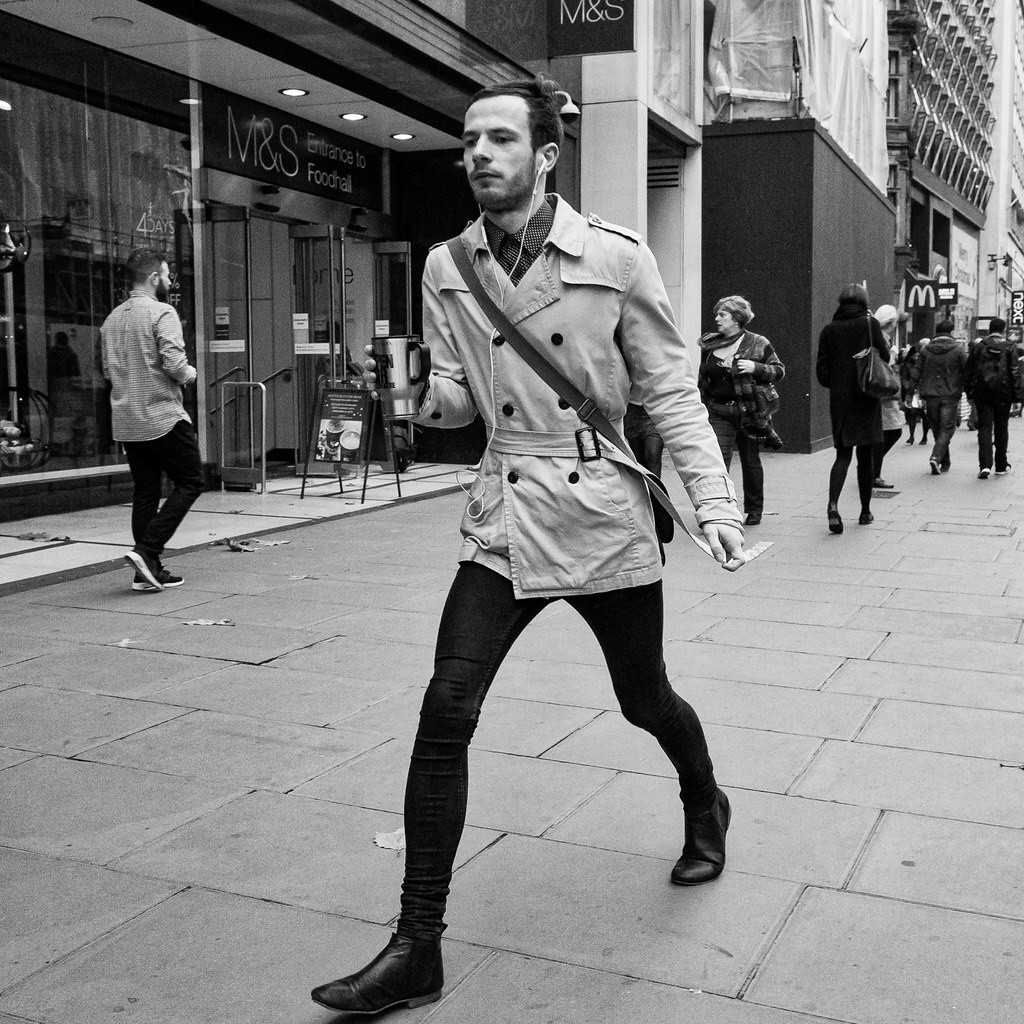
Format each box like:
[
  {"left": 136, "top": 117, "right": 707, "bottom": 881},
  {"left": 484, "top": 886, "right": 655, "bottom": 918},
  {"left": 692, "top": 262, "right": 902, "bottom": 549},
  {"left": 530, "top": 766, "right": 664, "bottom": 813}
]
[{"left": 537, "top": 157, "right": 548, "bottom": 176}]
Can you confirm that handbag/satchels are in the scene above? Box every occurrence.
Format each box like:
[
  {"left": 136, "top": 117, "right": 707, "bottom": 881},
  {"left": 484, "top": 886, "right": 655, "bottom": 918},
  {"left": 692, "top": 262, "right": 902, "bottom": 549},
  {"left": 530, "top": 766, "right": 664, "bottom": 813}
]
[
  {"left": 748, "top": 334, "right": 780, "bottom": 416},
  {"left": 852, "top": 316, "right": 900, "bottom": 400}
]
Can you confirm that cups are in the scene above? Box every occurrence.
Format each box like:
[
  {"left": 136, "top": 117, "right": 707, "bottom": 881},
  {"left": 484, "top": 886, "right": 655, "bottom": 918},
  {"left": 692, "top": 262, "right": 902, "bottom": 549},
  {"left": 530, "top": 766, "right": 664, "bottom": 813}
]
[
  {"left": 339, "top": 430, "right": 360, "bottom": 456},
  {"left": 325, "top": 422, "right": 345, "bottom": 454},
  {"left": 371, "top": 333, "right": 431, "bottom": 420}
]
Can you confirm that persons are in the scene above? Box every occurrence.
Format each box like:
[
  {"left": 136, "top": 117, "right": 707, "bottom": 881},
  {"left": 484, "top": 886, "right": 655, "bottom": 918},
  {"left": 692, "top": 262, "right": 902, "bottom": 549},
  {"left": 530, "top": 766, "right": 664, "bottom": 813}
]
[
  {"left": 696, "top": 295, "right": 785, "bottom": 528},
  {"left": 311, "top": 78, "right": 751, "bottom": 1016},
  {"left": 869, "top": 304, "right": 1021, "bottom": 495},
  {"left": 99, "top": 248, "right": 196, "bottom": 591},
  {"left": 815, "top": 282, "right": 893, "bottom": 534}
]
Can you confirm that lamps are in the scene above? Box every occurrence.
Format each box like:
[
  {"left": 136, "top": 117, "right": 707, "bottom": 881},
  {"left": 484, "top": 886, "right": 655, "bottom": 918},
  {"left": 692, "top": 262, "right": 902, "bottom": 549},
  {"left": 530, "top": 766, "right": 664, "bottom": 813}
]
[
  {"left": 348, "top": 223, "right": 370, "bottom": 233},
  {"left": 552, "top": 89, "right": 581, "bottom": 116},
  {"left": 1003, "top": 253, "right": 1012, "bottom": 267},
  {"left": 987, "top": 254, "right": 997, "bottom": 271},
  {"left": 254, "top": 202, "right": 280, "bottom": 214},
  {"left": 350, "top": 207, "right": 369, "bottom": 216},
  {"left": 259, "top": 184, "right": 280, "bottom": 196}
]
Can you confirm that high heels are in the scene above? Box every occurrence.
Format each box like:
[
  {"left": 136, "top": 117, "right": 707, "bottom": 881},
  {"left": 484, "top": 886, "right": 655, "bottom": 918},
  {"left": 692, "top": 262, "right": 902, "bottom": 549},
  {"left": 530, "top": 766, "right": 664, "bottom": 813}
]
[
  {"left": 919, "top": 439, "right": 927, "bottom": 445},
  {"left": 906, "top": 438, "right": 914, "bottom": 445}
]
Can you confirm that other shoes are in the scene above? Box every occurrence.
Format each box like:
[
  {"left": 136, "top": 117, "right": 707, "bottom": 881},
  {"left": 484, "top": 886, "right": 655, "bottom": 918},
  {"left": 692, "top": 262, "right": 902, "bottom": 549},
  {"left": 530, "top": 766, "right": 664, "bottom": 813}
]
[
  {"left": 941, "top": 465, "right": 949, "bottom": 471},
  {"left": 746, "top": 513, "right": 762, "bottom": 524},
  {"left": 995, "top": 464, "right": 1012, "bottom": 475},
  {"left": 859, "top": 512, "right": 874, "bottom": 524},
  {"left": 873, "top": 480, "right": 894, "bottom": 488},
  {"left": 978, "top": 466, "right": 991, "bottom": 478},
  {"left": 827, "top": 502, "right": 843, "bottom": 534},
  {"left": 930, "top": 457, "right": 940, "bottom": 475}
]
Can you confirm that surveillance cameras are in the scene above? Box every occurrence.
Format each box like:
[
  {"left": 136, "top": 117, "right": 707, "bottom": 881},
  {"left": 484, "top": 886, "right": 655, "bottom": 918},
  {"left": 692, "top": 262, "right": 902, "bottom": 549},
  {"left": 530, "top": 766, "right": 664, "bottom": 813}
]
[{"left": 559, "top": 103, "right": 582, "bottom": 124}]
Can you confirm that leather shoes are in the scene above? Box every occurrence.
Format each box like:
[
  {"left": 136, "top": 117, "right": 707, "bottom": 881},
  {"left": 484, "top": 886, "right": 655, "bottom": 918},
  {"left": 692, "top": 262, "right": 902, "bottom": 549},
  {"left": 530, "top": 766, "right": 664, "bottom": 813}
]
[
  {"left": 311, "top": 923, "right": 449, "bottom": 1013},
  {"left": 672, "top": 787, "right": 732, "bottom": 885}
]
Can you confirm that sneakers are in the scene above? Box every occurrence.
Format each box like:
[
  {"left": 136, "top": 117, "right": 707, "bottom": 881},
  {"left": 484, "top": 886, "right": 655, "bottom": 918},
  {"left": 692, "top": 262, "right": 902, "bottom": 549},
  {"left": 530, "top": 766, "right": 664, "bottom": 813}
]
[
  {"left": 124, "top": 550, "right": 165, "bottom": 591},
  {"left": 132, "top": 561, "right": 184, "bottom": 590}
]
